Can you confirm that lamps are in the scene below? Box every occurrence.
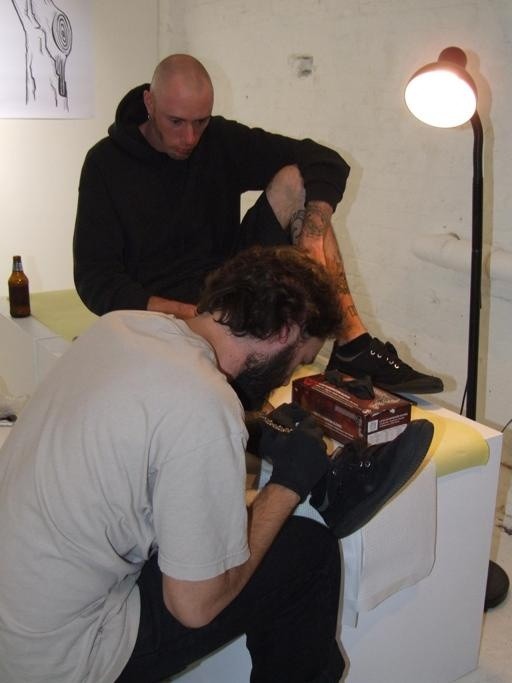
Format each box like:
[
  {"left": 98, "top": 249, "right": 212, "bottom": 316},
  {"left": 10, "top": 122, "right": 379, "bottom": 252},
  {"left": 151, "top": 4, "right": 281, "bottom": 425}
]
[{"left": 405, "top": 47, "right": 509, "bottom": 612}]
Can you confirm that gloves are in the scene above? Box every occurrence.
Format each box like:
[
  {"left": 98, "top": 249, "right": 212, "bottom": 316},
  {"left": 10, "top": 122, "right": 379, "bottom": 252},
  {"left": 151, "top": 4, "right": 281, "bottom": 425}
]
[
  {"left": 245, "top": 402, "right": 308, "bottom": 458},
  {"left": 266, "top": 415, "right": 329, "bottom": 505}
]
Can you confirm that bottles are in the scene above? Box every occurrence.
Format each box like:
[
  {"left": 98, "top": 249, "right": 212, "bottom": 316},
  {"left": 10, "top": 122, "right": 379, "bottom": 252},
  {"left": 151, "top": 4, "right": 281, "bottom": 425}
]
[{"left": 8, "top": 256, "right": 31, "bottom": 318}]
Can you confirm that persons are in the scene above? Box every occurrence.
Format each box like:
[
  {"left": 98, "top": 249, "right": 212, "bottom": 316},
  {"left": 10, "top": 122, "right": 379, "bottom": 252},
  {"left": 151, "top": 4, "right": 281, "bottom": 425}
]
[
  {"left": 71, "top": 53, "right": 445, "bottom": 395},
  {"left": 1, "top": 243, "right": 347, "bottom": 683}
]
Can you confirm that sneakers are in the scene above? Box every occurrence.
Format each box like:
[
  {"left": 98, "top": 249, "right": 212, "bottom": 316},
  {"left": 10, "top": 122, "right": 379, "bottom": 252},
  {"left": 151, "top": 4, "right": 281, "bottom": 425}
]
[
  {"left": 311, "top": 419, "right": 434, "bottom": 539},
  {"left": 324, "top": 337, "right": 444, "bottom": 393}
]
